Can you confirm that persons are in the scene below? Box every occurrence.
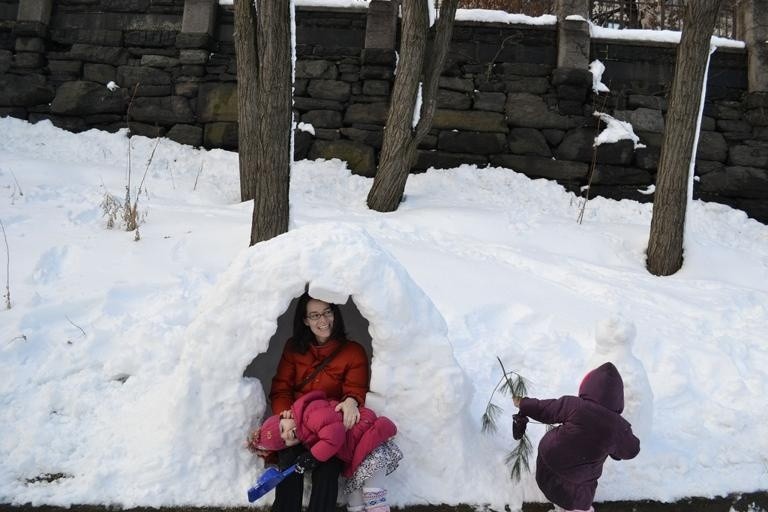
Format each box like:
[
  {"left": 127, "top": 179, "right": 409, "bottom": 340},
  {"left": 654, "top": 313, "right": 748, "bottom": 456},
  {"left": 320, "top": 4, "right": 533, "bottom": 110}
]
[
  {"left": 268, "top": 291, "right": 370, "bottom": 511},
  {"left": 511, "top": 360, "right": 640, "bottom": 511},
  {"left": 248, "top": 387, "right": 403, "bottom": 512}
]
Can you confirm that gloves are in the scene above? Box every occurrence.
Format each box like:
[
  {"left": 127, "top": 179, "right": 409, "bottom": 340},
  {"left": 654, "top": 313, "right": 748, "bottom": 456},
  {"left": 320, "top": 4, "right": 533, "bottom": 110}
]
[{"left": 294, "top": 452, "right": 320, "bottom": 475}]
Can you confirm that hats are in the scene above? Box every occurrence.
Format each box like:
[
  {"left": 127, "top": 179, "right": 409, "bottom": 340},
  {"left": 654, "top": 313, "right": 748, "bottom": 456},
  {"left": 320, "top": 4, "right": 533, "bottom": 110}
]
[{"left": 252, "top": 415, "right": 286, "bottom": 451}]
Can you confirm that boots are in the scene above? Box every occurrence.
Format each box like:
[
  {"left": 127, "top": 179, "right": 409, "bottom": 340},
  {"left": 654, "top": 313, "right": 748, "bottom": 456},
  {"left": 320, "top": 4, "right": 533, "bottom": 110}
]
[{"left": 346, "top": 502, "right": 390, "bottom": 512}]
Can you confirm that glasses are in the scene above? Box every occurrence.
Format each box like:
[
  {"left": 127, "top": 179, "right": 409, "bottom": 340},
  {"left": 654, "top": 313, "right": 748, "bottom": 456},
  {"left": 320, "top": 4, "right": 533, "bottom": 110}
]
[{"left": 305, "top": 310, "right": 332, "bottom": 319}]
